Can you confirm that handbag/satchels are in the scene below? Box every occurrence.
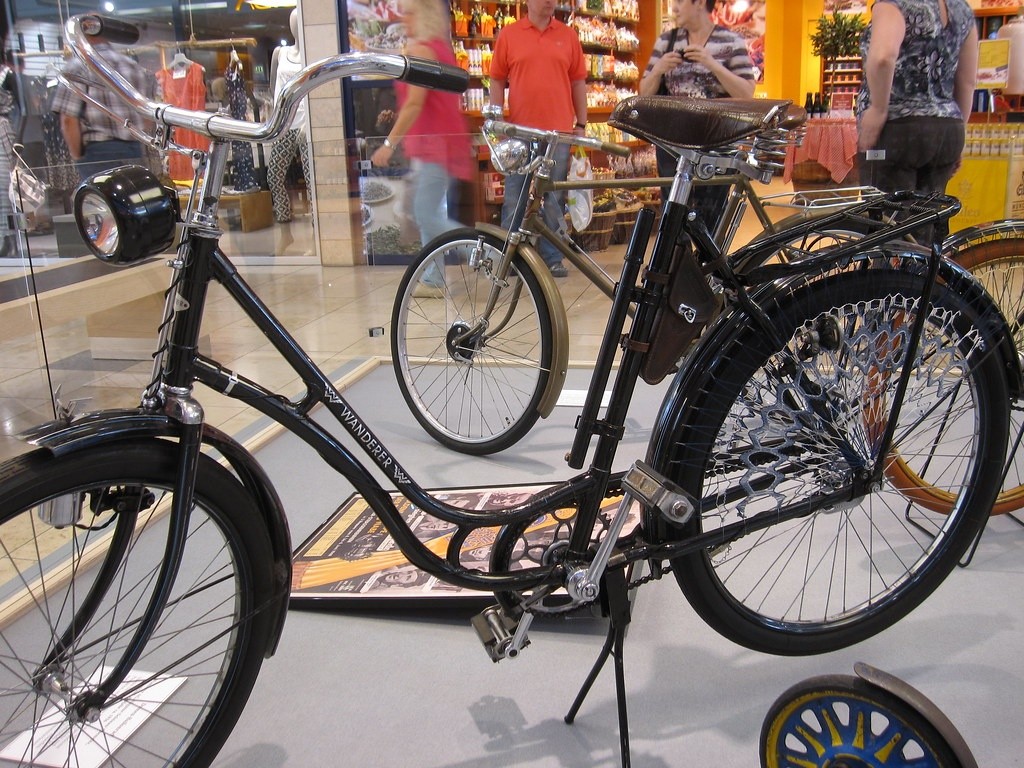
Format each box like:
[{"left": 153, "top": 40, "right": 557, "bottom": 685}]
[
  {"left": 656, "top": 74, "right": 671, "bottom": 95},
  {"left": 566, "top": 143, "right": 592, "bottom": 233}
]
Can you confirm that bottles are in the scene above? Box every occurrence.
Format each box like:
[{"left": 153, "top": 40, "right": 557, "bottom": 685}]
[
  {"left": 995, "top": 20, "right": 1024, "bottom": 95},
  {"left": 448, "top": 0, "right": 518, "bottom": 114},
  {"left": 812, "top": 92, "right": 822, "bottom": 119},
  {"left": 991, "top": 89, "right": 1005, "bottom": 114},
  {"left": 551, "top": 1, "right": 639, "bottom": 146},
  {"left": 804, "top": 92, "right": 813, "bottom": 119},
  {"left": 820, "top": 91, "right": 830, "bottom": 118}
]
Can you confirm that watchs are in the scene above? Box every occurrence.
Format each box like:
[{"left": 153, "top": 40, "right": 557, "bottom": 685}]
[{"left": 384, "top": 139, "right": 397, "bottom": 152}]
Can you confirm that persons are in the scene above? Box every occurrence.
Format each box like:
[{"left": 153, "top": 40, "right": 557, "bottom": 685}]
[
  {"left": 367, "top": 0, "right": 510, "bottom": 296},
  {"left": 0, "top": 42, "right": 27, "bottom": 255},
  {"left": 7, "top": 48, "right": 52, "bottom": 234},
  {"left": 48, "top": 15, "right": 162, "bottom": 176},
  {"left": 458, "top": 543, "right": 495, "bottom": 562},
  {"left": 856, "top": 1, "right": 978, "bottom": 273},
  {"left": 371, "top": 569, "right": 430, "bottom": 590},
  {"left": 486, "top": 0, "right": 589, "bottom": 281},
  {"left": 587, "top": 493, "right": 638, "bottom": 540},
  {"left": 268, "top": 7, "right": 317, "bottom": 259},
  {"left": 414, "top": 493, "right": 480, "bottom": 535},
  {"left": 484, "top": 492, "right": 540, "bottom": 511},
  {"left": 637, "top": 0, "right": 755, "bottom": 267}
]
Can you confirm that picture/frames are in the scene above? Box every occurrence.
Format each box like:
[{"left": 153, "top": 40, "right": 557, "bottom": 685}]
[{"left": 270, "top": 481, "right": 646, "bottom": 606}]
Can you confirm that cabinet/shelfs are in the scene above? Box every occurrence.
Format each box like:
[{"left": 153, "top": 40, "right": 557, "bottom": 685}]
[{"left": 442, "top": 0, "right": 666, "bottom": 219}]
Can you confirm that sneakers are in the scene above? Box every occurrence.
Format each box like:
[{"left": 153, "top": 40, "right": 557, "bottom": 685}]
[
  {"left": 410, "top": 280, "right": 444, "bottom": 299},
  {"left": 547, "top": 263, "right": 566, "bottom": 280}
]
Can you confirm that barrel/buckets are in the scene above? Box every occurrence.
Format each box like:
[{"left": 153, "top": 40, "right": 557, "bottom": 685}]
[
  {"left": 557, "top": 200, "right": 662, "bottom": 253},
  {"left": 786, "top": 115, "right": 860, "bottom": 204}
]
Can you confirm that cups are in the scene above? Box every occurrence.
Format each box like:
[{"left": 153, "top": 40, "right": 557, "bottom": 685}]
[
  {"left": 987, "top": 18, "right": 1000, "bottom": 39},
  {"left": 972, "top": 89, "right": 988, "bottom": 114},
  {"left": 824, "top": 54, "right": 862, "bottom": 94},
  {"left": 975, "top": 18, "right": 981, "bottom": 39},
  {"left": 961, "top": 122, "right": 1024, "bottom": 158}
]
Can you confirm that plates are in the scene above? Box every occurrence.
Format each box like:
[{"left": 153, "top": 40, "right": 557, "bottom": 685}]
[
  {"left": 360, "top": 179, "right": 394, "bottom": 205},
  {"left": 359, "top": 202, "right": 374, "bottom": 229},
  {"left": 393, "top": 200, "right": 415, "bottom": 222}
]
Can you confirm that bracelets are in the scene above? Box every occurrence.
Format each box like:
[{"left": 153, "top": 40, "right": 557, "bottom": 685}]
[{"left": 576, "top": 123, "right": 588, "bottom": 128}]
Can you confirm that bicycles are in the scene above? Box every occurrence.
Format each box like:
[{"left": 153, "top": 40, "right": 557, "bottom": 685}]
[
  {"left": 0, "top": 12, "right": 1023, "bottom": 768},
  {"left": 847, "top": 214, "right": 1024, "bottom": 570},
  {"left": 386, "top": 100, "right": 934, "bottom": 460},
  {"left": 758, "top": 659, "right": 980, "bottom": 768}
]
